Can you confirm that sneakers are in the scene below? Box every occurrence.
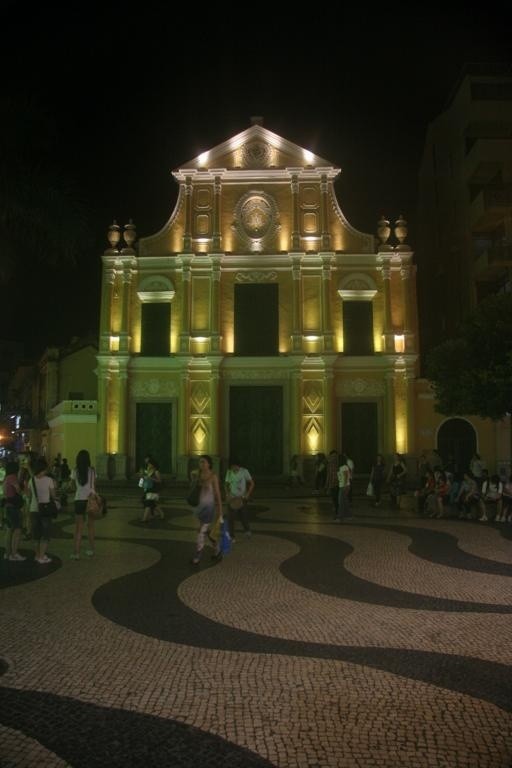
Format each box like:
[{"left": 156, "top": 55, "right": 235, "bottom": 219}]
[
  {"left": 191, "top": 557, "right": 201, "bottom": 568},
  {"left": 478, "top": 514, "right": 488, "bottom": 521},
  {"left": 245, "top": 530, "right": 251, "bottom": 537},
  {"left": 494, "top": 514, "right": 500, "bottom": 522},
  {"left": 69, "top": 554, "right": 80, "bottom": 562},
  {"left": 8, "top": 552, "right": 28, "bottom": 561},
  {"left": 3, "top": 552, "right": 9, "bottom": 560},
  {"left": 427, "top": 511, "right": 474, "bottom": 520},
  {"left": 38, "top": 556, "right": 52, "bottom": 565},
  {"left": 500, "top": 516, "right": 507, "bottom": 523},
  {"left": 84, "top": 549, "right": 95, "bottom": 556},
  {"left": 35, "top": 556, "right": 40, "bottom": 561}
]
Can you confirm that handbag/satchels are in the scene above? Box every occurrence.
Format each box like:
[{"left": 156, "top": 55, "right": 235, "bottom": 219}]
[
  {"left": 218, "top": 514, "right": 232, "bottom": 555},
  {"left": 186, "top": 469, "right": 203, "bottom": 508},
  {"left": 86, "top": 491, "right": 109, "bottom": 521},
  {"left": 38, "top": 500, "right": 59, "bottom": 519},
  {"left": 228, "top": 494, "right": 248, "bottom": 511}
]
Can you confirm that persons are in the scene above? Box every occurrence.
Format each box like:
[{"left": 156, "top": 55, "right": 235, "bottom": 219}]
[
  {"left": 141, "top": 459, "right": 166, "bottom": 522},
  {"left": 312, "top": 449, "right": 512, "bottom": 524},
  {"left": 286, "top": 453, "right": 304, "bottom": 488},
  {"left": 189, "top": 454, "right": 224, "bottom": 566},
  {"left": 143, "top": 456, "right": 156, "bottom": 520},
  {"left": 223, "top": 460, "right": 255, "bottom": 541},
  {"left": 0, "top": 440, "right": 99, "bottom": 565}
]
